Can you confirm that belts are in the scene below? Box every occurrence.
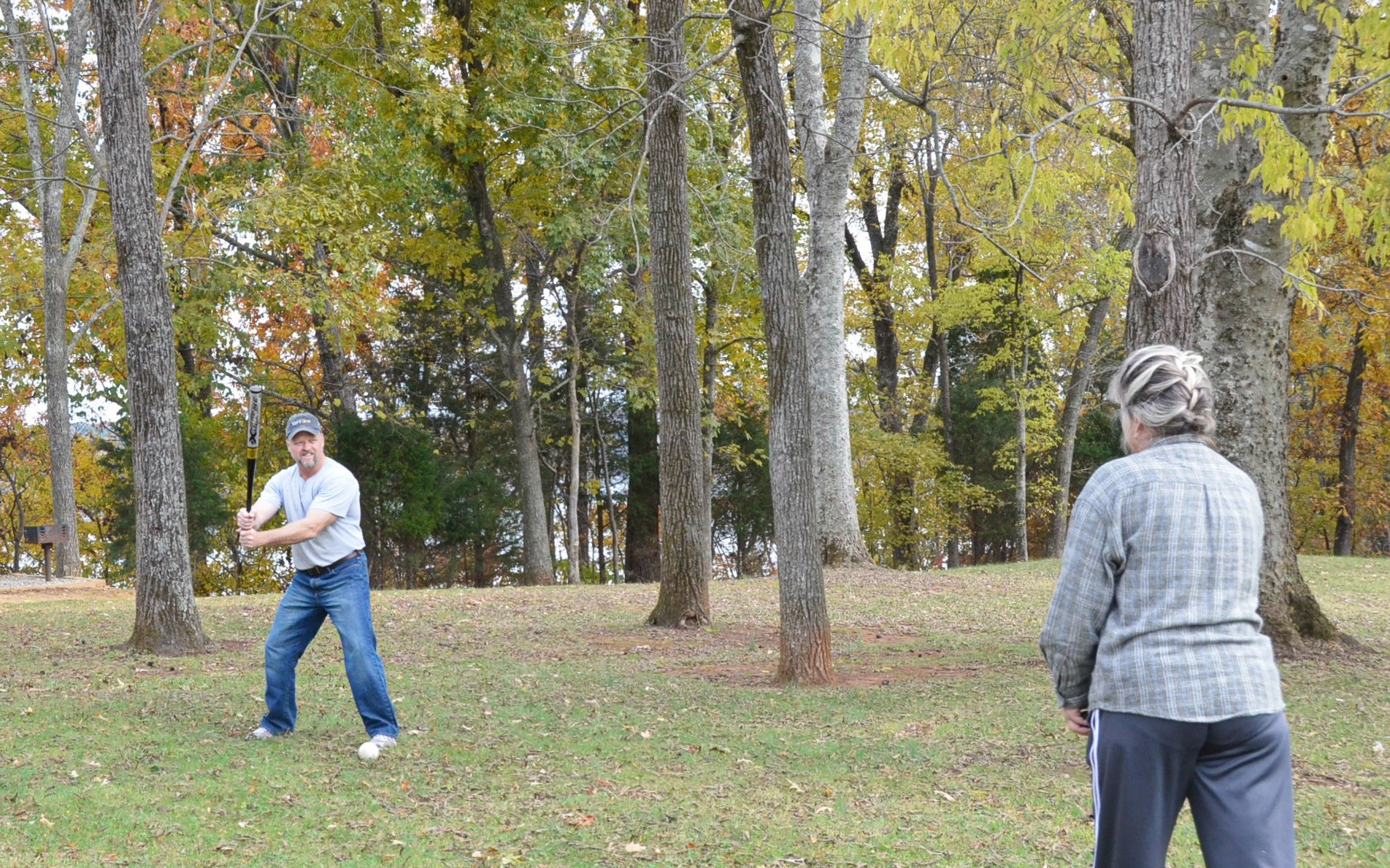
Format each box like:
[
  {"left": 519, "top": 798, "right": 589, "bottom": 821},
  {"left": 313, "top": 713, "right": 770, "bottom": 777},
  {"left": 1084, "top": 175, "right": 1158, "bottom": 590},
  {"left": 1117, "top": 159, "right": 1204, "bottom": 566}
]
[{"left": 300, "top": 549, "right": 365, "bottom": 575}]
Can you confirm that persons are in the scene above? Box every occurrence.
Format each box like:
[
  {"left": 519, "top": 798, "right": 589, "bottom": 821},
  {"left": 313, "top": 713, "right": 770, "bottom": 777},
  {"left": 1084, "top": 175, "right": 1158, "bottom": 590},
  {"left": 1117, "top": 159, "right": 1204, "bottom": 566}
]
[
  {"left": 1041, "top": 344, "right": 1296, "bottom": 868},
  {"left": 235, "top": 412, "right": 399, "bottom": 755}
]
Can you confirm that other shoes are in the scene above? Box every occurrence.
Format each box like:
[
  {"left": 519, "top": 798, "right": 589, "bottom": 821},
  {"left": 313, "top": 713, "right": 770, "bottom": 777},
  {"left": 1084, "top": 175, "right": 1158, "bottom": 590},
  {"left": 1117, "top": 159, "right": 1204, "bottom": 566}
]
[
  {"left": 370, "top": 735, "right": 398, "bottom": 749},
  {"left": 245, "top": 728, "right": 273, "bottom": 740}
]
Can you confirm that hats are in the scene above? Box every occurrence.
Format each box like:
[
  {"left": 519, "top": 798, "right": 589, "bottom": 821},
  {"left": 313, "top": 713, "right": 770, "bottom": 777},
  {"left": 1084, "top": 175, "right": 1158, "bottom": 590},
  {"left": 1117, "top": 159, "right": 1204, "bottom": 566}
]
[{"left": 285, "top": 412, "right": 322, "bottom": 441}]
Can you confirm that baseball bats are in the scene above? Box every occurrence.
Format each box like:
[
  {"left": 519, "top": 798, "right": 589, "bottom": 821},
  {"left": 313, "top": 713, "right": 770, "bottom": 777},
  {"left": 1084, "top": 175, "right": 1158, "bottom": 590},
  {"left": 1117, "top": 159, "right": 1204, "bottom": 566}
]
[{"left": 246, "top": 385, "right": 262, "bottom": 511}]
